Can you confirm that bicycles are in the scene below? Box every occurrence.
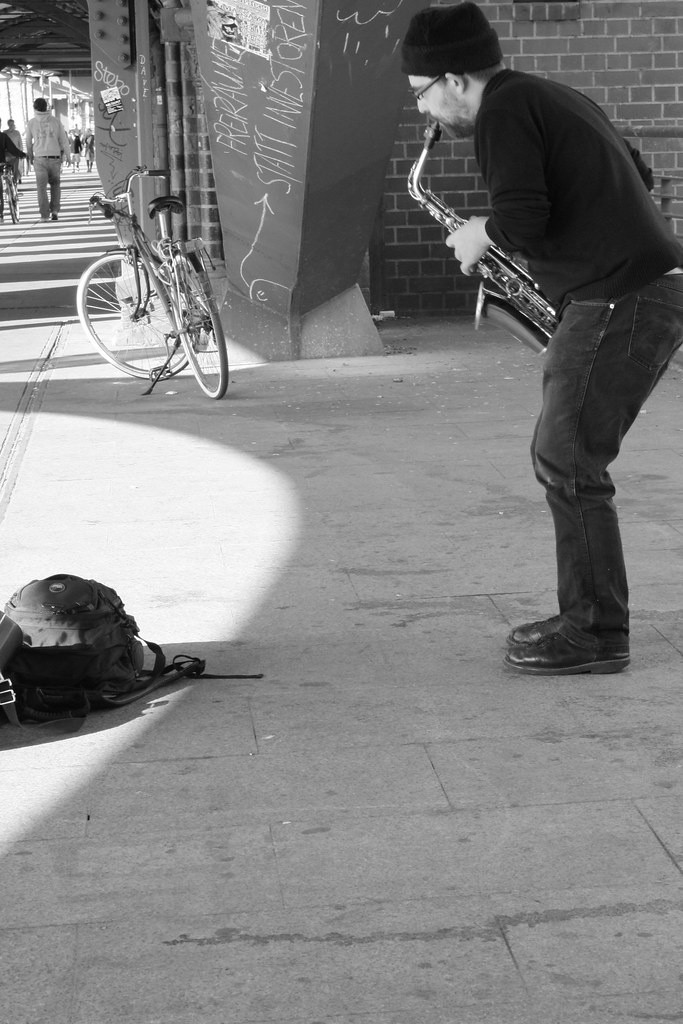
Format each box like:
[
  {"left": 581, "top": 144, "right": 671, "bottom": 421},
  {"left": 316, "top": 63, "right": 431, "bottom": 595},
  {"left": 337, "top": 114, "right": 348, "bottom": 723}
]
[
  {"left": 75, "top": 161, "right": 232, "bottom": 403},
  {"left": 0, "top": 154, "right": 29, "bottom": 224}
]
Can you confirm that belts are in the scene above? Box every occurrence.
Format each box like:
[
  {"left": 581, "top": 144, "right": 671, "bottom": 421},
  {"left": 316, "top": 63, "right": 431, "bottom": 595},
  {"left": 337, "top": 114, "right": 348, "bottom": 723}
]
[{"left": 38, "top": 156, "right": 61, "bottom": 159}]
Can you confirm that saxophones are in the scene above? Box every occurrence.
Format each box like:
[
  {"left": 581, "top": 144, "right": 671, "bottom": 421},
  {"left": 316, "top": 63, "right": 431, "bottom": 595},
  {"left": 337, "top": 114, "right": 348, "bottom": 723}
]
[{"left": 406, "top": 121, "right": 559, "bottom": 354}]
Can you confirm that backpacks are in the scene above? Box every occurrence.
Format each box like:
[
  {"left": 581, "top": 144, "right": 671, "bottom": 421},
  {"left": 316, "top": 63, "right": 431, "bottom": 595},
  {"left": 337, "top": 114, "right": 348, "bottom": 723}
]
[{"left": 3, "top": 573, "right": 206, "bottom": 732}]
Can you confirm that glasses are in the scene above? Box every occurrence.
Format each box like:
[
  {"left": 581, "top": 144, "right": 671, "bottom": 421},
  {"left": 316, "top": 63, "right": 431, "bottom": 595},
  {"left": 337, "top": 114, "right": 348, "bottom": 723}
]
[{"left": 408, "top": 73, "right": 465, "bottom": 99}]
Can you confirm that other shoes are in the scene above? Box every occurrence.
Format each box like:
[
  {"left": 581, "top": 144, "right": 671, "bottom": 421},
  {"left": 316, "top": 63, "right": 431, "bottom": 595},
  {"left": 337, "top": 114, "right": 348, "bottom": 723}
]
[
  {"left": 42, "top": 214, "right": 49, "bottom": 218},
  {"left": 51, "top": 211, "right": 58, "bottom": 220}
]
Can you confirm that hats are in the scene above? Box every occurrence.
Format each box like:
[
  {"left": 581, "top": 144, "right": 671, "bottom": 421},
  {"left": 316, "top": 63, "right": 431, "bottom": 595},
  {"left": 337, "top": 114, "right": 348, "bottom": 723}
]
[{"left": 402, "top": 2, "right": 503, "bottom": 75}]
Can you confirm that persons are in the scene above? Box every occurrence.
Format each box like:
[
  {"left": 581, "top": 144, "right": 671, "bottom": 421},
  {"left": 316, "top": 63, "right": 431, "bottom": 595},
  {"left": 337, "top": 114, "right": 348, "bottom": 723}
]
[
  {"left": 0, "top": 117, "right": 27, "bottom": 226},
  {"left": 63, "top": 122, "right": 95, "bottom": 173},
  {"left": 398, "top": 1, "right": 682, "bottom": 675},
  {"left": 25, "top": 97, "right": 73, "bottom": 223}
]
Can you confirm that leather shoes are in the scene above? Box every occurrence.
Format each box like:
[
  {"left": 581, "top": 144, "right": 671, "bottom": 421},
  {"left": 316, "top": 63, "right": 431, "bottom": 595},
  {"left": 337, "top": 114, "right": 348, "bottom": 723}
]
[
  {"left": 506, "top": 615, "right": 563, "bottom": 648},
  {"left": 503, "top": 633, "right": 631, "bottom": 676}
]
